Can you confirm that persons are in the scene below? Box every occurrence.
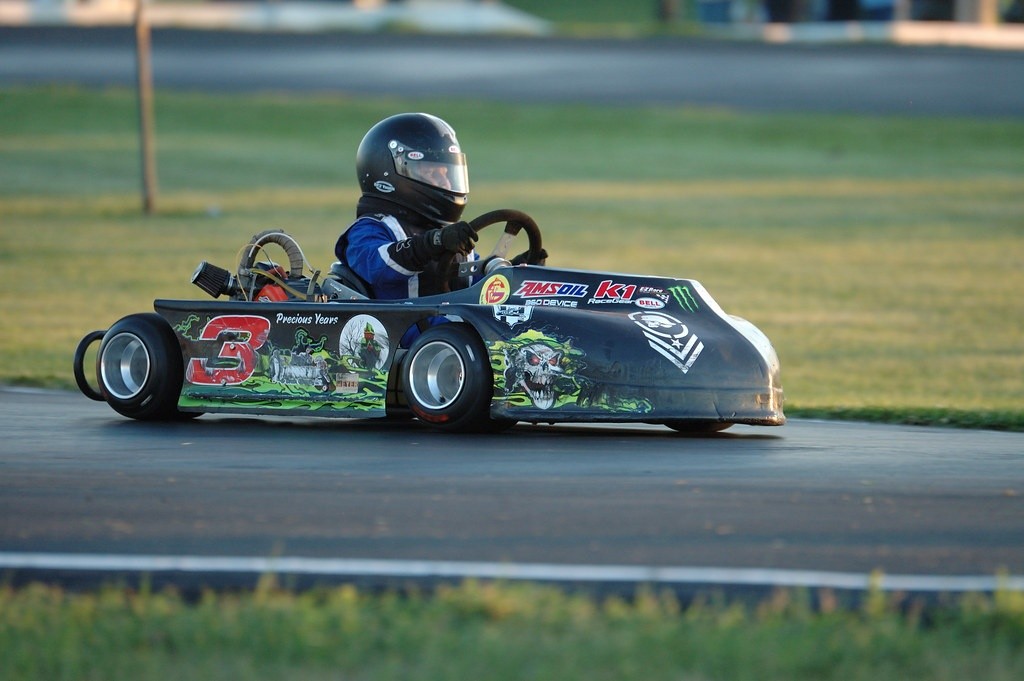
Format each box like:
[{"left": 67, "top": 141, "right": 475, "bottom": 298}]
[{"left": 333, "top": 113, "right": 547, "bottom": 300}]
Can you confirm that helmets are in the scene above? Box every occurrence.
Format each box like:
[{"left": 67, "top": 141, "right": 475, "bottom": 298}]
[{"left": 355, "top": 113, "right": 469, "bottom": 226}]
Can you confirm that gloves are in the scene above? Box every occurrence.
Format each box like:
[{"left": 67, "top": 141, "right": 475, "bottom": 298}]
[
  {"left": 412, "top": 221, "right": 478, "bottom": 267},
  {"left": 509, "top": 248, "right": 548, "bottom": 266}
]
